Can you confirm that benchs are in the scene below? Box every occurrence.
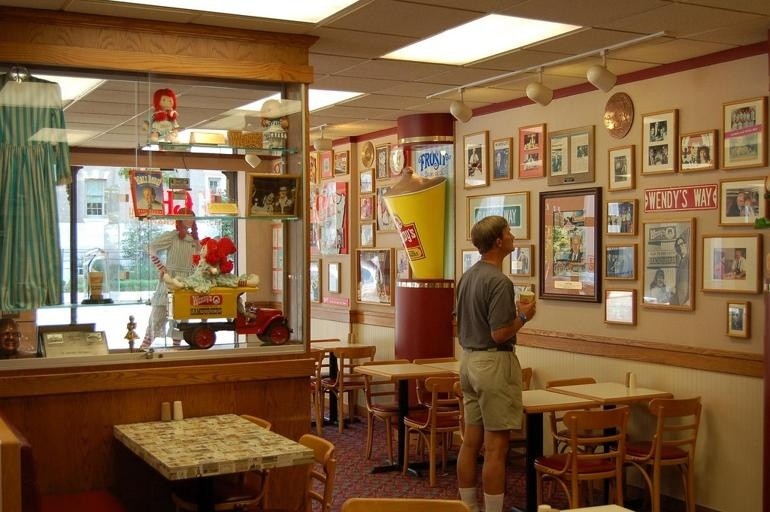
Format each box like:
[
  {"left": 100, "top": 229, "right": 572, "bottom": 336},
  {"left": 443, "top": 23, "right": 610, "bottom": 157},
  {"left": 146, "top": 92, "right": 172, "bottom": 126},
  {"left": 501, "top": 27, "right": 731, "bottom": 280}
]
[{"left": 1, "top": 411, "right": 132, "bottom": 512}]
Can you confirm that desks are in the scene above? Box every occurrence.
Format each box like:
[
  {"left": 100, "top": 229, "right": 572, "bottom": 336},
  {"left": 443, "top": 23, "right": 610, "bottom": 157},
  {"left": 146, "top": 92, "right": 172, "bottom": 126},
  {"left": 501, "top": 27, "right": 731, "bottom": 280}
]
[{"left": 112, "top": 412, "right": 315, "bottom": 511}]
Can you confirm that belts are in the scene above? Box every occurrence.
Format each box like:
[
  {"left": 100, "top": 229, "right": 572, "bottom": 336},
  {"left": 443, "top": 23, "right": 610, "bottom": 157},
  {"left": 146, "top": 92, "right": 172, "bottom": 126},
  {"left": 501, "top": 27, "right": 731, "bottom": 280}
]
[{"left": 462, "top": 346, "right": 515, "bottom": 353}]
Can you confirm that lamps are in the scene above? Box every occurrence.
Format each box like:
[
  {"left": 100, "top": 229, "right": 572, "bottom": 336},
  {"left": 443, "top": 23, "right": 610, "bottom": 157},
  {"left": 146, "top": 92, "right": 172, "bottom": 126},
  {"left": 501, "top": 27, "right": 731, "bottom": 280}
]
[{"left": 449, "top": 48, "right": 619, "bottom": 124}]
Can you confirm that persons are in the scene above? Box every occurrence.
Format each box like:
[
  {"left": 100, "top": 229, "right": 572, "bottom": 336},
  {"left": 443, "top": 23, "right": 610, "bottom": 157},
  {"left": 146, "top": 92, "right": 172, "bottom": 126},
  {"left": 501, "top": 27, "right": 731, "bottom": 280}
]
[
  {"left": 732, "top": 250, "right": 746, "bottom": 280},
  {"left": 649, "top": 125, "right": 668, "bottom": 164},
  {"left": 650, "top": 270, "right": 672, "bottom": 304},
  {"left": 609, "top": 210, "right": 633, "bottom": 232},
  {"left": 568, "top": 238, "right": 581, "bottom": 264},
  {"left": 672, "top": 237, "right": 690, "bottom": 305},
  {"left": 251, "top": 183, "right": 296, "bottom": 216},
  {"left": 470, "top": 149, "right": 479, "bottom": 163},
  {"left": 149, "top": 88, "right": 181, "bottom": 143},
  {"left": 731, "top": 309, "right": 739, "bottom": 331},
  {"left": 714, "top": 252, "right": 726, "bottom": 279},
  {"left": 685, "top": 135, "right": 709, "bottom": 165},
  {"left": 139, "top": 208, "right": 259, "bottom": 349},
  {"left": 470, "top": 163, "right": 480, "bottom": 178},
  {"left": 728, "top": 192, "right": 755, "bottom": 217},
  {"left": 495, "top": 151, "right": 505, "bottom": 176},
  {"left": 732, "top": 109, "right": 756, "bottom": 129},
  {"left": 136, "top": 185, "right": 164, "bottom": 211},
  {"left": 456, "top": 215, "right": 537, "bottom": 512}
]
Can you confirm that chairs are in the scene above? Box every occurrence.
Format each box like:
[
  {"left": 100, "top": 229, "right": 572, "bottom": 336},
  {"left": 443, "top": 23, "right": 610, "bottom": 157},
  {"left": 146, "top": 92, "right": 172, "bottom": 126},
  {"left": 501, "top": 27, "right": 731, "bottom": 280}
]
[
  {"left": 353, "top": 358, "right": 532, "bottom": 487},
  {"left": 507, "top": 373, "right": 702, "bottom": 510},
  {"left": 307, "top": 344, "right": 377, "bottom": 431},
  {"left": 259, "top": 432, "right": 336, "bottom": 511},
  {"left": 170, "top": 414, "right": 273, "bottom": 511},
  {"left": 343, "top": 496, "right": 470, "bottom": 511}
]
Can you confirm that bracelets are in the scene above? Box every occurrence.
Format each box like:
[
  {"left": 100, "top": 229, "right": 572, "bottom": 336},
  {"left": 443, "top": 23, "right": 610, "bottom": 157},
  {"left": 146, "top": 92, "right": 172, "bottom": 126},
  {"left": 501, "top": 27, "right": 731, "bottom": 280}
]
[{"left": 518, "top": 312, "right": 527, "bottom": 324}]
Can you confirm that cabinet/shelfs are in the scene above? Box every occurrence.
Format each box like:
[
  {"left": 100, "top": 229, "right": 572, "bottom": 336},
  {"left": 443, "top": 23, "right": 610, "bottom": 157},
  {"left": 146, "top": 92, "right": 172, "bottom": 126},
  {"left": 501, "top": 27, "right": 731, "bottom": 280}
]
[{"left": 133, "top": 140, "right": 303, "bottom": 224}]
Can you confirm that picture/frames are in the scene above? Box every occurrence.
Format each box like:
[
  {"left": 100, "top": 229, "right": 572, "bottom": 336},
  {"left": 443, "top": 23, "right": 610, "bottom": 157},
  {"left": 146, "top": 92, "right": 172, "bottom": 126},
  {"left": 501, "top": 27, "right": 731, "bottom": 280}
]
[
  {"left": 310, "top": 138, "right": 405, "bottom": 309},
  {"left": 547, "top": 124, "right": 595, "bottom": 186},
  {"left": 640, "top": 216, "right": 697, "bottom": 312},
  {"left": 245, "top": 172, "right": 302, "bottom": 221},
  {"left": 608, "top": 145, "right": 636, "bottom": 192},
  {"left": 604, "top": 198, "right": 638, "bottom": 237},
  {"left": 510, "top": 243, "right": 534, "bottom": 277},
  {"left": 466, "top": 191, "right": 531, "bottom": 240},
  {"left": 492, "top": 137, "right": 513, "bottom": 181},
  {"left": 678, "top": 129, "right": 719, "bottom": 173},
  {"left": 518, "top": 124, "right": 548, "bottom": 179},
  {"left": 461, "top": 248, "right": 484, "bottom": 274},
  {"left": 604, "top": 287, "right": 638, "bottom": 326},
  {"left": 722, "top": 97, "right": 769, "bottom": 171},
  {"left": 718, "top": 175, "right": 770, "bottom": 226},
  {"left": 701, "top": 231, "right": 763, "bottom": 295},
  {"left": 463, "top": 130, "right": 491, "bottom": 189},
  {"left": 605, "top": 243, "right": 639, "bottom": 282},
  {"left": 725, "top": 299, "right": 751, "bottom": 339},
  {"left": 539, "top": 186, "right": 603, "bottom": 304},
  {"left": 512, "top": 282, "right": 535, "bottom": 317},
  {"left": 640, "top": 108, "right": 680, "bottom": 175}
]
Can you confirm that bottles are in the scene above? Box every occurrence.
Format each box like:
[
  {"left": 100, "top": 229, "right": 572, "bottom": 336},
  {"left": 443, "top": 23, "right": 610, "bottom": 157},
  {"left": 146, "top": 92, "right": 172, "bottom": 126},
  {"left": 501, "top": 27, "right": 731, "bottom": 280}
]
[
  {"left": 160, "top": 401, "right": 172, "bottom": 421},
  {"left": 623, "top": 371, "right": 639, "bottom": 390},
  {"left": 173, "top": 400, "right": 184, "bottom": 420},
  {"left": 262, "top": 117, "right": 287, "bottom": 151}
]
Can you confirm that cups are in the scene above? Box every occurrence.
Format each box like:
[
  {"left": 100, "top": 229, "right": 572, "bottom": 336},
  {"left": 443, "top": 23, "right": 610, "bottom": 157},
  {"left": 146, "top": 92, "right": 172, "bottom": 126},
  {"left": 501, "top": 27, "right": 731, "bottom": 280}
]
[
  {"left": 517, "top": 292, "right": 535, "bottom": 315},
  {"left": 87, "top": 271, "right": 104, "bottom": 300}
]
